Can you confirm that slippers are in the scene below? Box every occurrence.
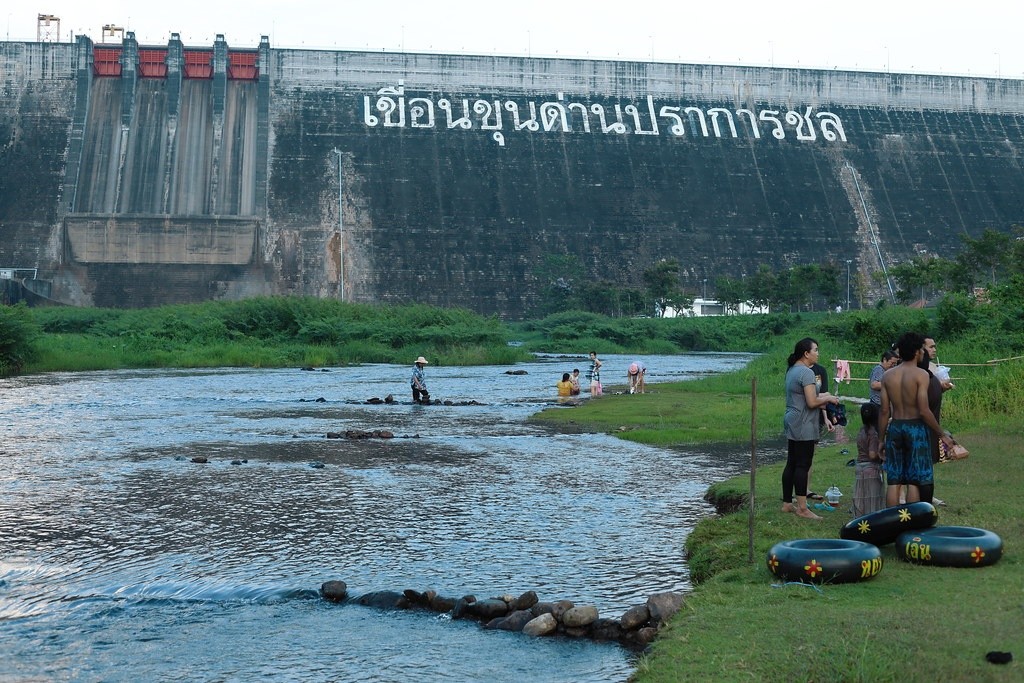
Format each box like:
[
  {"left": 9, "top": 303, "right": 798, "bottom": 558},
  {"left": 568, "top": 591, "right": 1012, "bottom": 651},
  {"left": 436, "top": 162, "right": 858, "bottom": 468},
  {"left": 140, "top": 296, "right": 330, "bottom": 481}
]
[
  {"left": 807, "top": 491, "right": 823, "bottom": 500},
  {"left": 814, "top": 501, "right": 835, "bottom": 511}
]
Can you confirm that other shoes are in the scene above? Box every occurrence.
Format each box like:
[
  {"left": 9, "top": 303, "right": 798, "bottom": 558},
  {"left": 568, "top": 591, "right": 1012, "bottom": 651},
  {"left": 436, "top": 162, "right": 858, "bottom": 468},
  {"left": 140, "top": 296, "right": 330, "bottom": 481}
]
[
  {"left": 899, "top": 497, "right": 906, "bottom": 504},
  {"left": 932, "top": 497, "right": 946, "bottom": 506}
]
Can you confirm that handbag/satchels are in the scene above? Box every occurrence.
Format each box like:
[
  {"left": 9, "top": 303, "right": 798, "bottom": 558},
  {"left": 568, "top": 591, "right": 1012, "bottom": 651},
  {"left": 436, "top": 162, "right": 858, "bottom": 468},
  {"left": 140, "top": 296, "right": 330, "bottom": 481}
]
[{"left": 938, "top": 436, "right": 969, "bottom": 462}]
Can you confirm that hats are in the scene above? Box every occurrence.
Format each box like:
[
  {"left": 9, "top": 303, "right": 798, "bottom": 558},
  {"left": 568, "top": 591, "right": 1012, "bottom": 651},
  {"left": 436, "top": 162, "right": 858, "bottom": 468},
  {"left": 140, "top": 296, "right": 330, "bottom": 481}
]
[
  {"left": 629, "top": 363, "right": 639, "bottom": 375},
  {"left": 414, "top": 356, "right": 429, "bottom": 364}
]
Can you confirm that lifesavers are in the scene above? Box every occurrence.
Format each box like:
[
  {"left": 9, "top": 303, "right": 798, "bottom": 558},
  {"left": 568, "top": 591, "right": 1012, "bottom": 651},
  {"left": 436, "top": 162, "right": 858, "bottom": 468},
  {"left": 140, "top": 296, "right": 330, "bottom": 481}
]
[
  {"left": 766, "top": 538, "right": 883, "bottom": 586},
  {"left": 840, "top": 501, "right": 938, "bottom": 547},
  {"left": 895, "top": 525, "right": 1003, "bottom": 568}
]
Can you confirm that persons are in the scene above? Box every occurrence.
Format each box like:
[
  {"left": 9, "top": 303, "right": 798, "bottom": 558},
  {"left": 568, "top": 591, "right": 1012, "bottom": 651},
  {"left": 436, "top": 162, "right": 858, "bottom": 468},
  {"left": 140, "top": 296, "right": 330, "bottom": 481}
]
[
  {"left": 921, "top": 335, "right": 953, "bottom": 506},
  {"left": 781, "top": 338, "right": 837, "bottom": 520},
  {"left": 585, "top": 351, "right": 602, "bottom": 398},
  {"left": 627, "top": 361, "right": 646, "bottom": 394},
  {"left": 410, "top": 356, "right": 429, "bottom": 400},
  {"left": 557, "top": 374, "right": 578, "bottom": 397},
  {"left": 892, "top": 347, "right": 948, "bottom": 504},
  {"left": 852, "top": 403, "right": 884, "bottom": 520},
  {"left": 866, "top": 351, "right": 898, "bottom": 406},
  {"left": 569, "top": 369, "right": 580, "bottom": 395},
  {"left": 876, "top": 331, "right": 954, "bottom": 508},
  {"left": 780, "top": 363, "right": 835, "bottom": 503}
]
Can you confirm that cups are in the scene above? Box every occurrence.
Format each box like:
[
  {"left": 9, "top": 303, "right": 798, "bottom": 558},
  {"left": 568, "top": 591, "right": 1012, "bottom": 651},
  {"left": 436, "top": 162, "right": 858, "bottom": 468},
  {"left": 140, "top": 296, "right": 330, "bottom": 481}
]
[
  {"left": 828, "top": 487, "right": 840, "bottom": 507},
  {"left": 934, "top": 366, "right": 950, "bottom": 386}
]
